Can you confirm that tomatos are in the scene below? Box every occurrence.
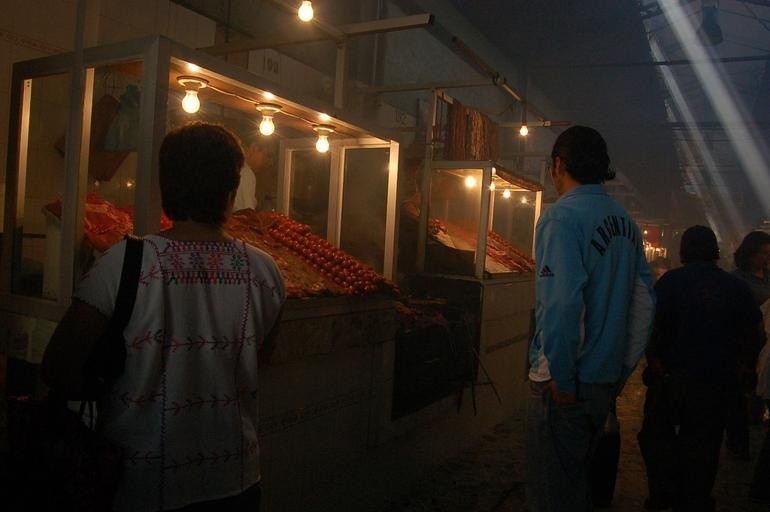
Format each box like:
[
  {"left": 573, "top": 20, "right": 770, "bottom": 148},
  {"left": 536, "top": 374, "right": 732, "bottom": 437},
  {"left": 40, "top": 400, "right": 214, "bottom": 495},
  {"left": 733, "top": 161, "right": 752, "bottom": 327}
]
[{"left": 258, "top": 210, "right": 396, "bottom": 296}]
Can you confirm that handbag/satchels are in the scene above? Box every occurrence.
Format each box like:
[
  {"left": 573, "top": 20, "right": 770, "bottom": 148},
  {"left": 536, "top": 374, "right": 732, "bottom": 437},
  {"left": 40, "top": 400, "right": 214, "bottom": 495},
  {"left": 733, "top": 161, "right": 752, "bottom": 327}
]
[{"left": 63, "top": 328, "right": 127, "bottom": 401}]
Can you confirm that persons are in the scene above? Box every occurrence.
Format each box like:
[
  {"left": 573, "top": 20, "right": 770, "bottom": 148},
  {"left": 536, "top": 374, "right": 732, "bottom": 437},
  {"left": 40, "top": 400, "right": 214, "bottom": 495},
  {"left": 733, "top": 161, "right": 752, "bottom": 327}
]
[
  {"left": 731, "top": 229, "right": 770, "bottom": 306},
  {"left": 42, "top": 119, "right": 296, "bottom": 512},
  {"left": 234, "top": 134, "right": 267, "bottom": 215},
  {"left": 401, "top": 190, "right": 423, "bottom": 216},
  {"left": 636, "top": 223, "right": 768, "bottom": 511},
  {"left": 723, "top": 398, "right": 751, "bottom": 458},
  {"left": 523, "top": 123, "right": 657, "bottom": 511}
]
